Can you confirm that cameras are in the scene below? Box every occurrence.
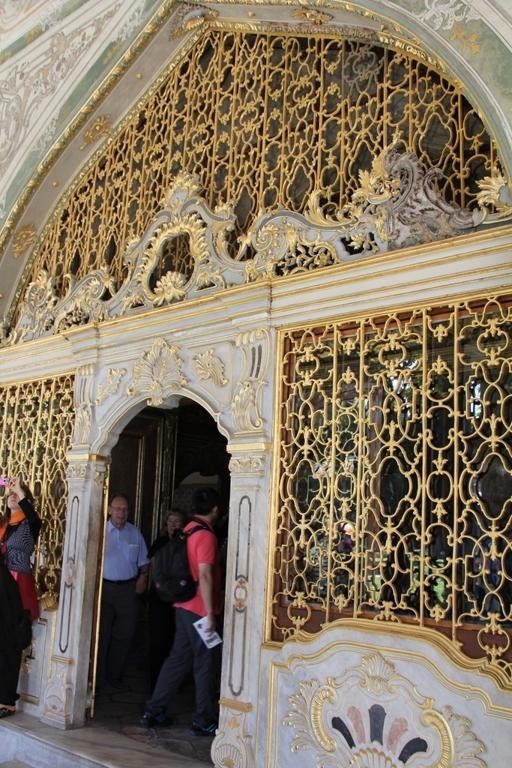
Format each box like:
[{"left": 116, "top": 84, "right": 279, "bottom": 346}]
[{"left": 0, "top": 477, "right": 10, "bottom": 486}]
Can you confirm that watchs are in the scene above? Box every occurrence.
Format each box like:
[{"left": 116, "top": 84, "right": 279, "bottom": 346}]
[{"left": 139, "top": 571, "right": 149, "bottom": 577}]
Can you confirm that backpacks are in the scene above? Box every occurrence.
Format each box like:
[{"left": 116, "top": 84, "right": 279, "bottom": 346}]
[{"left": 148, "top": 524, "right": 212, "bottom": 609}]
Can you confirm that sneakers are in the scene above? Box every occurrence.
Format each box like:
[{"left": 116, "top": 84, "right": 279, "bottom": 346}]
[
  {"left": 0, "top": 708, "right": 16, "bottom": 718},
  {"left": 141, "top": 714, "right": 172, "bottom": 727},
  {"left": 190, "top": 722, "right": 218, "bottom": 736}
]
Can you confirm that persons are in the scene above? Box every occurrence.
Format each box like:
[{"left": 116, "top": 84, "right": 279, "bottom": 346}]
[
  {"left": 146, "top": 508, "right": 189, "bottom": 694},
  {"left": 92, "top": 492, "right": 151, "bottom": 687},
  {"left": 138, "top": 488, "right": 224, "bottom": 738},
  {"left": 0, "top": 476, "right": 44, "bottom": 719}
]
